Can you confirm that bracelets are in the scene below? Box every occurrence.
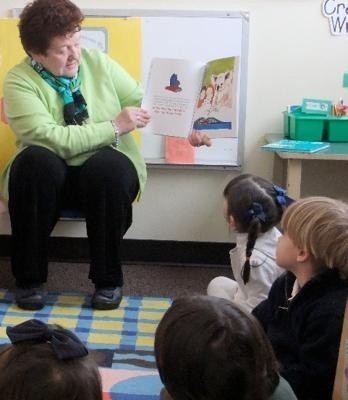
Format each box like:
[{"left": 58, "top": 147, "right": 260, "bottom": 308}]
[{"left": 110, "top": 119, "right": 119, "bottom": 148}]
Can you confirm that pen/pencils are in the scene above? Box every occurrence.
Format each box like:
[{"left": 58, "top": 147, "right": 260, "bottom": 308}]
[{"left": 334, "top": 99, "right": 346, "bottom": 117}]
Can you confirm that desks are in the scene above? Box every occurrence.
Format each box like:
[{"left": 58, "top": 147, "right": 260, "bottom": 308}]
[{"left": 265, "top": 132, "right": 348, "bottom": 201}]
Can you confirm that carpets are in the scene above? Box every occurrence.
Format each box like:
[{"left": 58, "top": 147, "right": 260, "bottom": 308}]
[{"left": 0, "top": 288, "right": 173, "bottom": 400}]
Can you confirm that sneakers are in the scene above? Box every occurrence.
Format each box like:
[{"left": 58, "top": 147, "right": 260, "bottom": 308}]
[
  {"left": 90, "top": 286, "right": 123, "bottom": 310},
  {"left": 14, "top": 283, "right": 49, "bottom": 311}
]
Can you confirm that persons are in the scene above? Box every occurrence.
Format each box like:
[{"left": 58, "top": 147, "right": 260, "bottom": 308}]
[
  {"left": 249, "top": 195, "right": 348, "bottom": 400},
  {"left": 207, "top": 171, "right": 296, "bottom": 313},
  {"left": 0, "top": 0, "right": 216, "bottom": 311},
  {"left": 1, "top": 318, "right": 104, "bottom": 400},
  {"left": 153, "top": 291, "right": 297, "bottom": 400}
]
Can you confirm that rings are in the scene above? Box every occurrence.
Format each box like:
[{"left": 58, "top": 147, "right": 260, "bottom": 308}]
[{"left": 195, "top": 143, "right": 201, "bottom": 147}]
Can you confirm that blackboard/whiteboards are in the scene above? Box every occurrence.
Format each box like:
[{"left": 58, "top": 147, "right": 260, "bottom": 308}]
[{"left": 10, "top": 7, "right": 249, "bottom": 171}]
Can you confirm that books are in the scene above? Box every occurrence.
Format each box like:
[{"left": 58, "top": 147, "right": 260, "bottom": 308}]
[
  {"left": 138, "top": 54, "right": 239, "bottom": 139},
  {"left": 259, "top": 139, "right": 329, "bottom": 153}
]
